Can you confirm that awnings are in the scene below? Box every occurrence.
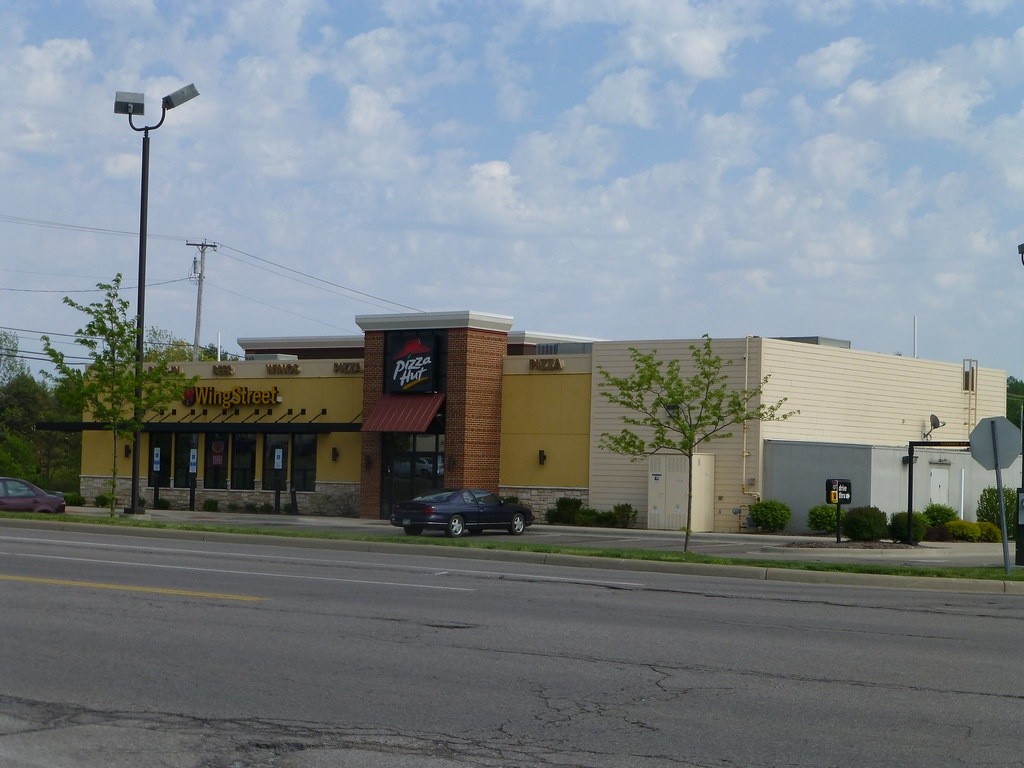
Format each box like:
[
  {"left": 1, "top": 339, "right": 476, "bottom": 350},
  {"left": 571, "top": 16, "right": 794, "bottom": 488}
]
[{"left": 360, "top": 394, "right": 445, "bottom": 432}]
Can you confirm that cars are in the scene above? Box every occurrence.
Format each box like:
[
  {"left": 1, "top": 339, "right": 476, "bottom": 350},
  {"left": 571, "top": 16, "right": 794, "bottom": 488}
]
[
  {"left": 389, "top": 486, "right": 537, "bottom": 539},
  {"left": 0, "top": 476, "right": 66, "bottom": 516}
]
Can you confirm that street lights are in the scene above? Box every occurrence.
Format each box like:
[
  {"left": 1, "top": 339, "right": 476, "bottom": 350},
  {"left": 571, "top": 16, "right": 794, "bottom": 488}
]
[{"left": 114, "top": 84, "right": 202, "bottom": 515}]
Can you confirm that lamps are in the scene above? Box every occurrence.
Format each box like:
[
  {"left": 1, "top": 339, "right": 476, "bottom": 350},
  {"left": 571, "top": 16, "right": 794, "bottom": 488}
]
[
  {"left": 146, "top": 409, "right": 326, "bottom": 425},
  {"left": 539, "top": 449, "right": 547, "bottom": 464},
  {"left": 364, "top": 455, "right": 372, "bottom": 470},
  {"left": 125, "top": 445, "right": 131, "bottom": 457},
  {"left": 331, "top": 447, "right": 339, "bottom": 461},
  {"left": 448, "top": 456, "right": 456, "bottom": 470}
]
